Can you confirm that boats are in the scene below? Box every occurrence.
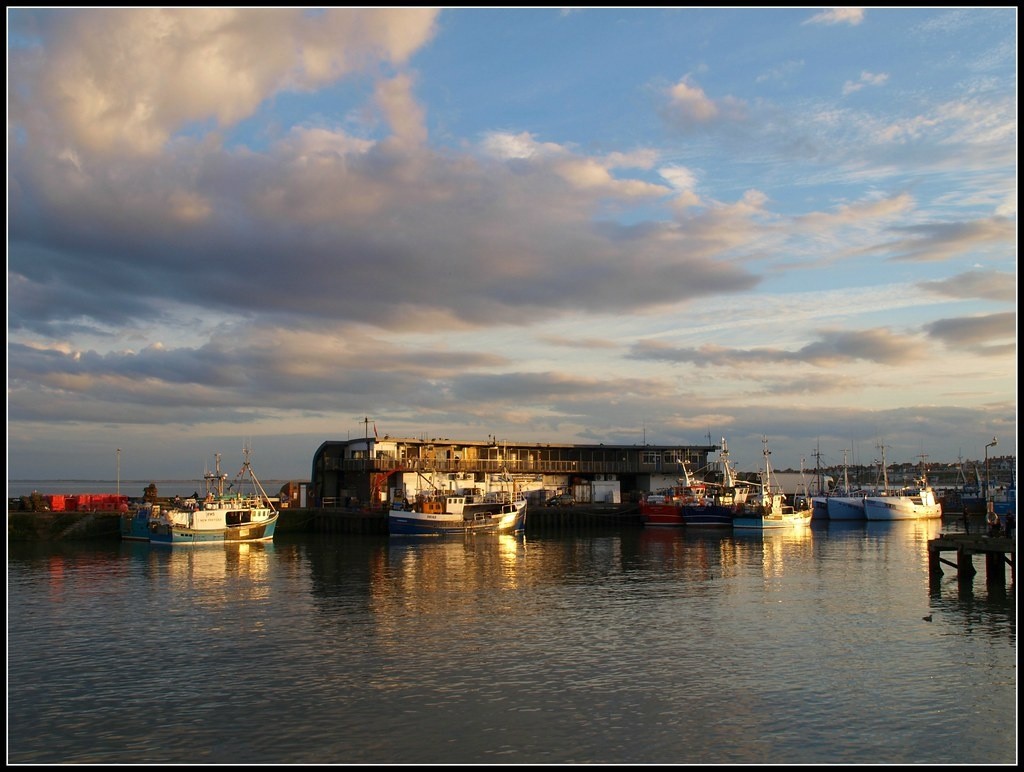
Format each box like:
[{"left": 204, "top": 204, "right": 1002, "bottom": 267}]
[{"left": 121, "top": 434, "right": 1015, "bottom": 546}]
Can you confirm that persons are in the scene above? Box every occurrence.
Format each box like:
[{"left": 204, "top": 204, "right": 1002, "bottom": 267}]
[
  {"left": 174, "top": 494, "right": 181, "bottom": 509},
  {"left": 1005, "top": 510, "right": 1014, "bottom": 538},
  {"left": 1000, "top": 485, "right": 1004, "bottom": 490},
  {"left": 959, "top": 504, "right": 970, "bottom": 535},
  {"left": 191, "top": 491, "right": 199, "bottom": 498}
]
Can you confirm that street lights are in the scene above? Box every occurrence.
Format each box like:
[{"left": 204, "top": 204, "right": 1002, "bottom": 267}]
[{"left": 117, "top": 449, "right": 120, "bottom": 495}]
